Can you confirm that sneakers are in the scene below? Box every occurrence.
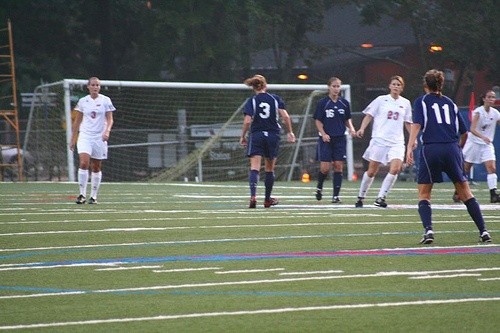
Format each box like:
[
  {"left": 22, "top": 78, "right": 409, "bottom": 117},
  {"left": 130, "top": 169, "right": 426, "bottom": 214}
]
[
  {"left": 332, "top": 196, "right": 343, "bottom": 203},
  {"left": 76, "top": 195, "right": 86, "bottom": 203},
  {"left": 479, "top": 233, "right": 491, "bottom": 242},
  {"left": 88, "top": 197, "right": 97, "bottom": 204},
  {"left": 355, "top": 196, "right": 365, "bottom": 208},
  {"left": 374, "top": 197, "right": 387, "bottom": 207},
  {"left": 250, "top": 196, "right": 256, "bottom": 208},
  {"left": 453, "top": 190, "right": 461, "bottom": 202},
  {"left": 316, "top": 188, "right": 322, "bottom": 200},
  {"left": 491, "top": 194, "right": 500, "bottom": 203},
  {"left": 264, "top": 198, "right": 278, "bottom": 208},
  {"left": 420, "top": 233, "right": 434, "bottom": 244}
]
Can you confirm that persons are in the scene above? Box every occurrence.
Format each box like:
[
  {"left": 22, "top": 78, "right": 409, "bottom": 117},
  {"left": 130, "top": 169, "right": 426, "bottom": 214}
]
[
  {"left": 69, "top": 77, "right": 116, "bottom": 204},
  {"left": 312, "top": 77, "right": 357, "bottom": 203},
  {"left": 452, "top": 90, "right": 500, "bottom": 203},
  {"left": 406, "top": 69, "right": 492, "bottom": 245},
  {"left": 355, "top": 76, "right": 418, "bottom": 208},
  {"left": 240, "top": 75, "right": 296, "bottom": 209}
]
